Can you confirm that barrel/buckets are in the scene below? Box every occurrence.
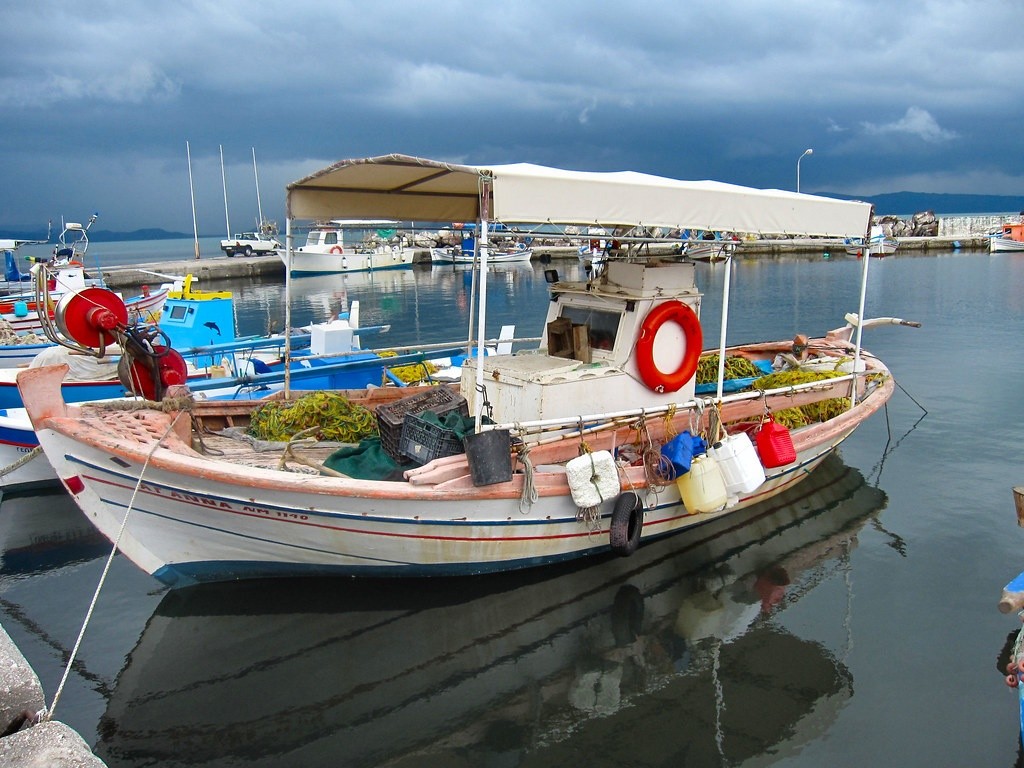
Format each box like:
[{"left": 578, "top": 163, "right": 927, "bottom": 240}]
[
  {"left": 676, "top": 454, "right": 728, "bottom": 513},
  {"left": 461, "top": 428, "right": 515, "bottom": 486},
  {"left": 754, "top": 413, "right": 798, "bottom": 469}
]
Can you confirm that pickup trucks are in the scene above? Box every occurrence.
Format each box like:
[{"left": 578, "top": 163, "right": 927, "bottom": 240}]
[{"left": 219, "top": 232, "right": 280, "bottom": 257}]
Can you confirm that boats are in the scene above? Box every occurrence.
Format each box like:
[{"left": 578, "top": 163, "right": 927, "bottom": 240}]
[
  {"left": 988, "top": 223, "right": 1022, "bottom": 255},
  {"left": 2, "top": 149, "right": 898, "bottom": 588}
]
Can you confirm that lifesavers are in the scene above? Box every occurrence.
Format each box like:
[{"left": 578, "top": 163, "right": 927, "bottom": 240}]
[
  {"left": 637, "top": 300, "right": 703, "bottom": 393},
  {"left": 330, "top": 246, "right": 343, "bottom": 254}
]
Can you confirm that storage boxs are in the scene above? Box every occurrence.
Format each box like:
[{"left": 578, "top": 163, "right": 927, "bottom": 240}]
[
  {"left": 373, "top": 384, "right": 471, "bottom": 466},
  {"left": 398, "top": 412, "right": 465, "bottom": 466}
]
